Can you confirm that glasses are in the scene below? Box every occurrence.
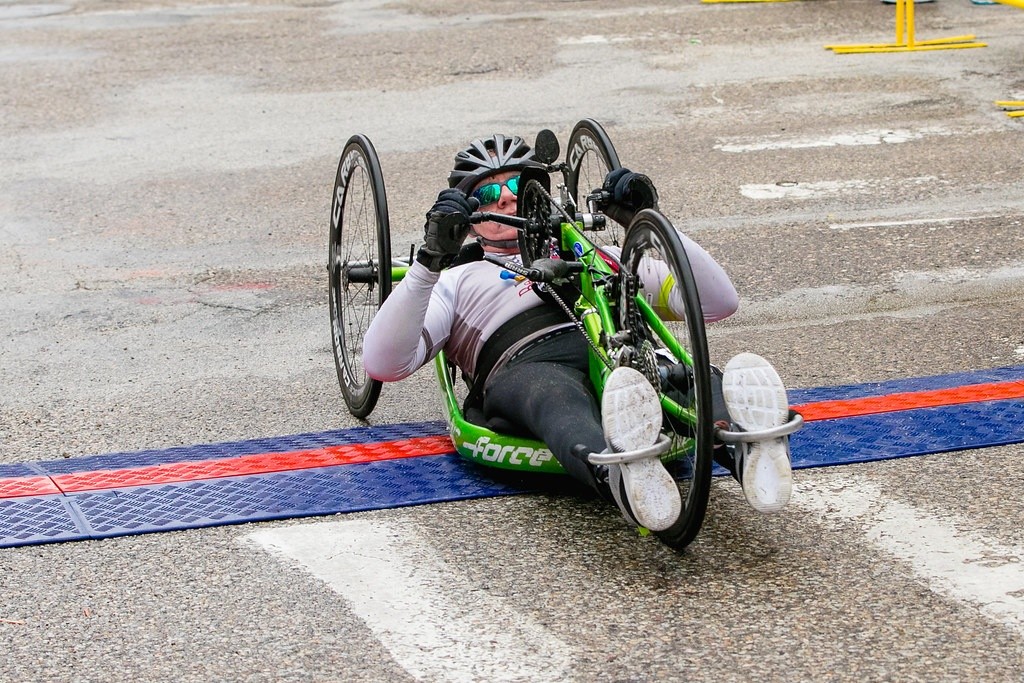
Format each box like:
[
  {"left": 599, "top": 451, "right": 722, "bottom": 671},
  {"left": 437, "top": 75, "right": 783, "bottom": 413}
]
[{"left": 472, "top": 175, "right": 521, "bottom": 208}]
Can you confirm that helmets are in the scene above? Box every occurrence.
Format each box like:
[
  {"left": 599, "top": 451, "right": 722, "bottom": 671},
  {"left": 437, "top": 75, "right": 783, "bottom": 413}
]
[{"left": 447, "top": 134, "right": 547, "bottom": 194}]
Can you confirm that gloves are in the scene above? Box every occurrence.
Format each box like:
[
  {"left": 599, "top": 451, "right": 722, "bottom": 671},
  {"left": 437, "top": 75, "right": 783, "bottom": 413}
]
[
  {"left": 424, "top": 188, "right": 480, "bottom": 254},
  {"left": 590, "top": 167, "right": 659, "bottom": 226}
]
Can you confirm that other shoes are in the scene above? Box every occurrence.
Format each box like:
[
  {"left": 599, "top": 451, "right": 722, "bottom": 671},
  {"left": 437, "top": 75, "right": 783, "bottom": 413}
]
[
  {"left": 599, "top": 367, "right": 682, "bottom": 537},
  {"left": 721, "top": 350, "right": 793, "bottom": 514}
]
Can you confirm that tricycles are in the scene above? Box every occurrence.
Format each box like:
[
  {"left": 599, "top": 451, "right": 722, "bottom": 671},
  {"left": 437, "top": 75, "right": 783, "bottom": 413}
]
[{"left": 326, "top": 118, "right": 806, "bottom": 552}]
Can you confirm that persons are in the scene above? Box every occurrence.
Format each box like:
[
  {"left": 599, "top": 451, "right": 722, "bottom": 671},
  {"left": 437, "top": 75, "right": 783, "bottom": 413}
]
[{"left": 364, "top": 133, "right": 793, "bottom": 530}]
